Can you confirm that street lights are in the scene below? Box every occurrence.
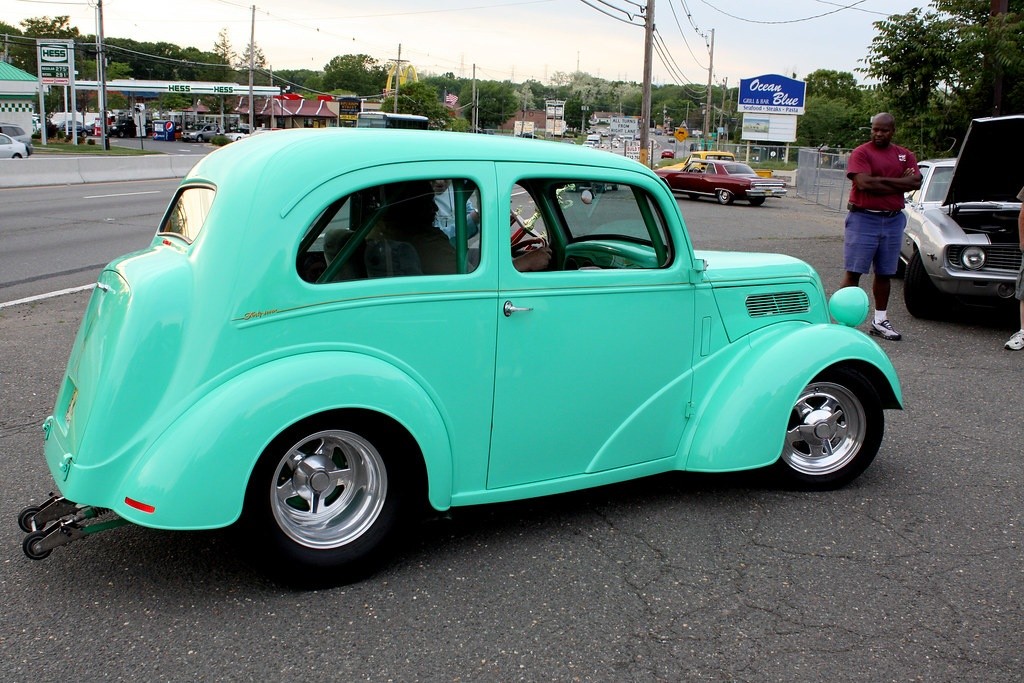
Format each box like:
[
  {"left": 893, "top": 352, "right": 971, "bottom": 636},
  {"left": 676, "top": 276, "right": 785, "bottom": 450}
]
[{"left": 700, "top": 103, "right": 715, "bottom": 150}]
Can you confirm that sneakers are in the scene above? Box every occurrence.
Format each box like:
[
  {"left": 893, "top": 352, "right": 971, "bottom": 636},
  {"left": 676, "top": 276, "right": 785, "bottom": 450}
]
[
  {"left": 1004, "top": 330, "right": 1024, "bottom": 351},
  {"left": 869, "top": 319, "right": 902, "bottom": 341}
]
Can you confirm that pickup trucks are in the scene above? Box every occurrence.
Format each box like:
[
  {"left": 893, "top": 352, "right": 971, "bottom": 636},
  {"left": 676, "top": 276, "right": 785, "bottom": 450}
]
[{"left": 657, "top": 150, "right": 773, "bottom": 179}]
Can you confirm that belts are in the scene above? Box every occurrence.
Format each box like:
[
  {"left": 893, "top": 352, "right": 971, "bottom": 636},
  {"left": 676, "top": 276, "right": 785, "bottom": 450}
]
[{"left": 854, "top": 206, "right": 900, "bottom": 218}]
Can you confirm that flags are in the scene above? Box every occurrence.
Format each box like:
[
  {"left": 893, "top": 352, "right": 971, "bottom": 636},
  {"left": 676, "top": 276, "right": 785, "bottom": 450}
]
[{"left": 446, "top": 90, "right": 458, "bottom": 105}]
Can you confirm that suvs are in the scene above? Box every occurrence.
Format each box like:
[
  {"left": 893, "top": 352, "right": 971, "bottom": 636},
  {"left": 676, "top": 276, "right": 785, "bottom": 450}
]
[{"left": 107, "top": 118, "right": 152, "bottom": 138}]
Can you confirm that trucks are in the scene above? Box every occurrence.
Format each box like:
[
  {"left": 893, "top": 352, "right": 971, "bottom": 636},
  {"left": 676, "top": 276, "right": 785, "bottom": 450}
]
[{"left": 587, "top": 135, "right": 603, "bottom": 148}]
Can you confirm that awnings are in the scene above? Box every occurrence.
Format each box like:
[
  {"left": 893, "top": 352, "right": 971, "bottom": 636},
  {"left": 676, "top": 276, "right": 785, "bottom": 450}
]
[{"left": 231, "top": 96, "right": 337, "bottom": 117}]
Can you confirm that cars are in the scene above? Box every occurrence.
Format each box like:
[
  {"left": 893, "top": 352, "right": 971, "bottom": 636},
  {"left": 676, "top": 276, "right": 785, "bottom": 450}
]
[
  {"left": 180, "top": 124, "right": 225, "bottom": 142},
  {"left": 586, "top": 128, "right": 662, "bottom": 147},
  {"left": 896, "top": 113, "right": 1024, "bottom": 321},
  {"left": 667, "top": 130, "right": 673, "bottom": 136},
  {"left": 18, "top": 123, "right": 905, "bottom": 583},
  {"left": 0, "top": 123, "right": 34, "bottom": 159},
  {"left": 668, "top": 138, "right": 676, "bottom": 143},
  {"left": 56, "top": 120, "right": 93, "bottom": 138},
  {"left": 581, "top": 141, "right": 596, "bottom": 149},
  {"left": 660, "top": 149, "right": 675, "bottom": 159},
  {"left": 834, "top": 161, "right": 845, "bottom": 169},
  {"left": 216, "top": 126, "right": 281, "bottom": 142},
  {"left": 520, "top": 133, "right": 537, "bottom": 139},
  {"left": 569, "top": 182, "right": 622, "bottom": 194},
  {"left": 651, "top": 159, "right": 787, "bottom": 206}
]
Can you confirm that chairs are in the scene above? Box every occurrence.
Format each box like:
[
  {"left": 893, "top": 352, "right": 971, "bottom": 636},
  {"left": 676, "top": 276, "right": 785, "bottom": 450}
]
[{"left": 323, "top": 229, "right": 425, "bottom": 281}]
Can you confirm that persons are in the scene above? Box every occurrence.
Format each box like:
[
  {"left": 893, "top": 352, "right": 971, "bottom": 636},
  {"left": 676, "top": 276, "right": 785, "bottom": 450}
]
[
  {"left": 432, "top": 179, "right": 480, "bottom": 250},
  {"left": 839, "top": 112, "right": 923, "bottom": 341},
  {"left": 380, "top": 180, "right": 552, "bottom": 275},
  {"left": 1003, "top": 186, "right": 1024, "bottom": 350}
]
